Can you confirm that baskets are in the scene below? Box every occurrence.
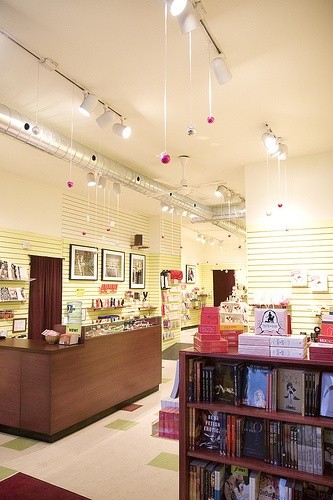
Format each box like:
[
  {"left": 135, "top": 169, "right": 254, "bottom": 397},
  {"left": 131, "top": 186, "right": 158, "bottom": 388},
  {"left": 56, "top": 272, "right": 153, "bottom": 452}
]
[{"left": 45, "top": 335, "right": 59, "bottom": 343}]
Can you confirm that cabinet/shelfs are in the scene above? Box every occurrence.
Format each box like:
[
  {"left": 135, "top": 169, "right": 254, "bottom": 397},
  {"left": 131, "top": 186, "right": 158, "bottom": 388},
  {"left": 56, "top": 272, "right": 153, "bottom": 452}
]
[
  {"left": 179, "top": 346, "right": 333, "bottom": 500},
  {"left": 0, "top": 279, "right": 28, "bottom": 302},
  {"left": 219, "top": 302, "right": 249, "bottom": 325}
]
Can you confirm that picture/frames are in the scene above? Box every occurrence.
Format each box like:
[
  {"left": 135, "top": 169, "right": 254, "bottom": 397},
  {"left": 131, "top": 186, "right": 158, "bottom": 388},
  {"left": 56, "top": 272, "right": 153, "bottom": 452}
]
[
  {"left": 186, "top": 265, "right": 196, "bottom": 284},
  {"left": 102, "top": 248, "right": 124, "bottom": 281},
  {"left": 129, "top": 253, "right": 144, "bottom": 289},
  {"left": 69, "top": 243, "right": 98, "bottom": 281}
]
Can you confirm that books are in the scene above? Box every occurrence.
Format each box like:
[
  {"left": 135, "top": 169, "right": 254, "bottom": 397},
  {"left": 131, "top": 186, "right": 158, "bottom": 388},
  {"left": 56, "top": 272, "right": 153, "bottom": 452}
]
[{"left": 0, "top": 260, "right": 28, "bottom": 301}]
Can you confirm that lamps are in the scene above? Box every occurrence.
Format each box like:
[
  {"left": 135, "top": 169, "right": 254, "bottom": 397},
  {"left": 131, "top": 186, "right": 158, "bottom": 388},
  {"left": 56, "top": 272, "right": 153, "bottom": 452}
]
[{"left": 70, "top": 0, "right": 287, "bottom": 249}]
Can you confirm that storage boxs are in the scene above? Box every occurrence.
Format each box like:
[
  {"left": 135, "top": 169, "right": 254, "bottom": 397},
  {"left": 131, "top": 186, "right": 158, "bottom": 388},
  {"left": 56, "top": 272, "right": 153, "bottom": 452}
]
[{"left": 159, "top": 396, "right": 179, "bottom": 439}]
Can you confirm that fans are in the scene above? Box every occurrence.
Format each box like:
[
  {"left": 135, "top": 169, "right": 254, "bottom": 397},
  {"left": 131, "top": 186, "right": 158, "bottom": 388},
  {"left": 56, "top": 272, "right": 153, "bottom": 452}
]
[{"left": 146, "top": 155, "right": 225, "bottom": 196}]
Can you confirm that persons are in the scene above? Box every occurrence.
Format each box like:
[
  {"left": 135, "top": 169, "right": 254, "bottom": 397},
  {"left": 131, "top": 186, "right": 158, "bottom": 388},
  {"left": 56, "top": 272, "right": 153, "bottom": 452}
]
[{"left": 188, "top": 358, "right": 333, "bottom": 500}]
